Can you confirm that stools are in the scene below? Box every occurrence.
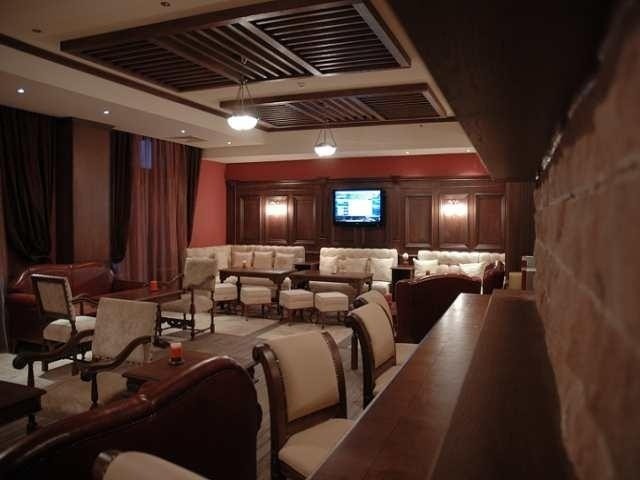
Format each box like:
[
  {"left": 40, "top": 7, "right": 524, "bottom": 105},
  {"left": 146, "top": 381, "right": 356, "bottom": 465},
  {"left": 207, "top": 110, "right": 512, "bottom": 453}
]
[{"left": 213, "top": 284, "right": 348, "bottom": 330}]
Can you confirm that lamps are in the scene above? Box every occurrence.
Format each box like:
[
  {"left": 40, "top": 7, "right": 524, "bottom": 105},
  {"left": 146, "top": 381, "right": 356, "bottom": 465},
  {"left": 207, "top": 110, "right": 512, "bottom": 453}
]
[
  {"left": 226, "top": 71, "right": 259, "bottom": 131},
  {"left": 313, "top": 117, "right": 337, "bottom": 155}
]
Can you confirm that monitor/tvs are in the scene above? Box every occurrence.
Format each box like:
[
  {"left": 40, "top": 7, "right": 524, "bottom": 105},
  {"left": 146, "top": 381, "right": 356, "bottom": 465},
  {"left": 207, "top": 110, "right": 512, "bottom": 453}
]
[{"left": 332, "top": 188, "right": 384, "bottom": 227}]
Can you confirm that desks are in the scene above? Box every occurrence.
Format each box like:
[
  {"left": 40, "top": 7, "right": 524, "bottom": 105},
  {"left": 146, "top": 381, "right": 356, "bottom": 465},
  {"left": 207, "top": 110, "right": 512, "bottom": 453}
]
[
  {"left": 305, "top": 293, "right": 574, "bottom": 479},
  {"left": 87, "top": 284, "right": 185, "bottom": 348},
  {"left": 0, "top": 380, "right": 48, "bottom": 439},
  {"left": 217, "top": 267, "right": 375, "bottom": 301}
]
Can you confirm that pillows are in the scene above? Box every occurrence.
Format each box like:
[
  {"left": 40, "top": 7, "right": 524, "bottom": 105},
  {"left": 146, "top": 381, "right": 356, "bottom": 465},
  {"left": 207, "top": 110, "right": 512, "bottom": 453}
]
[
  {"left": 213, "top": 249, "right": 296, "bottom": 273},
  {"left": 412, "top": 256, "right": 484, "bottom": 277},
  {"left": 318, "top": 254, "right": 394, "bottom": 281}
]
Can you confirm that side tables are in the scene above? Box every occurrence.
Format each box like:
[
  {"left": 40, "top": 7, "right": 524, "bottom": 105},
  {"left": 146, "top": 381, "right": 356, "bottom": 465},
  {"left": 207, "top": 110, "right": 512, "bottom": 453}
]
[
  {"left": 121, "top": 350, "right": 259, "bottom": 399},
  {"left": 293, "top": 260, "right": 319, "bottom": 272},
  {"left": 391, "top": 263, "right": 417, "bottom": 299}
]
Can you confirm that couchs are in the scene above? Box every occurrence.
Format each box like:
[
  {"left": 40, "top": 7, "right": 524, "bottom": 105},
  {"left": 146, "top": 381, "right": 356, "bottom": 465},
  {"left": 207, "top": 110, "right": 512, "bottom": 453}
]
[
  {"left": 7, "top": 259, "right": 143, "bottom": 355},
  {"left": 183, "top": 244, "right": 306, "bottom": 283},
  {"left": 318, "top": 246, "right": 398, "bottom": 298},
  {"left": 396, "top": 272, "right": 484, "bottom": 342},
  {"left": 411, "top": 249, "right": 506, "bottom": 284}
]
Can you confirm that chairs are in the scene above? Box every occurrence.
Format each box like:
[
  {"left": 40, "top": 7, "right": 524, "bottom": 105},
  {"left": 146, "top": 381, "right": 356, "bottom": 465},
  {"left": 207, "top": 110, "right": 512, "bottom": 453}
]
[
  {"left": 162, "top": 256, "right": 217, "bottom": 341},
  {"left": 480, "top": 260, "right": 504, "bottom": 294},
  {"left": 249, "top": 290, "right": 413, "bottom": 479},
  {"left": 12, "top": 272, "right": 161, "bottom": 436}
]
